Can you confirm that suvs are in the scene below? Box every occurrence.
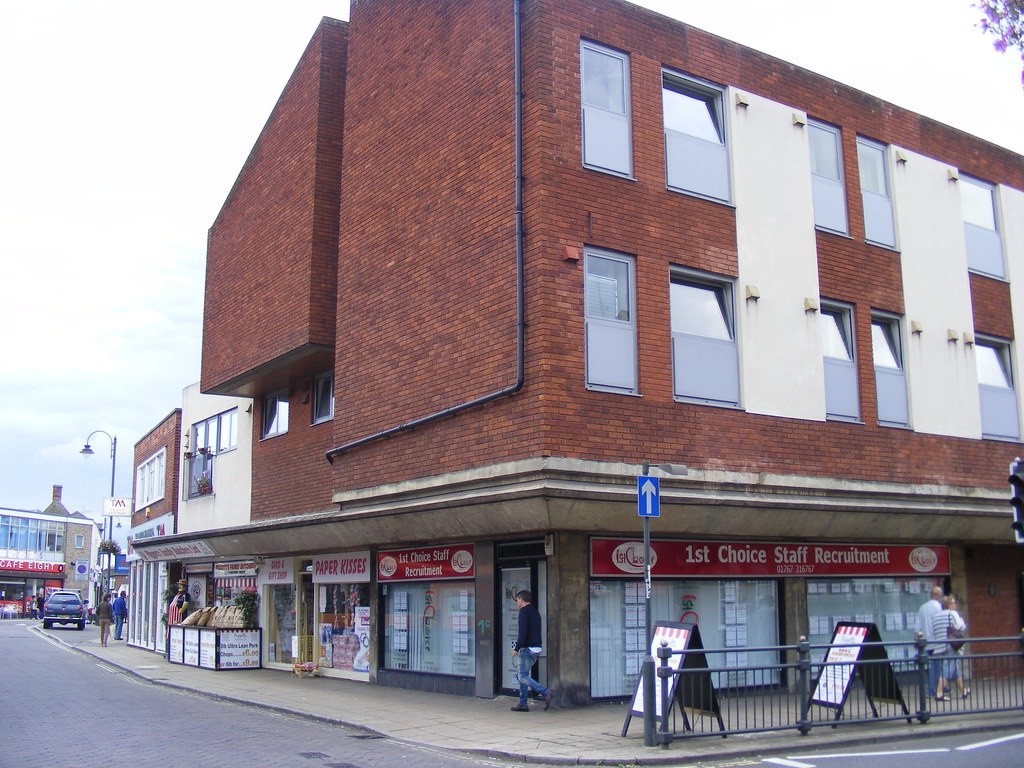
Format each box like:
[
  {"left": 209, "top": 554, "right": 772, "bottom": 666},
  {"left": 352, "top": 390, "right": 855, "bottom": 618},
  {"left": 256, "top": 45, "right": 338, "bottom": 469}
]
[{"left": 43, "top": 591, "right": 89, "bottom": 631}]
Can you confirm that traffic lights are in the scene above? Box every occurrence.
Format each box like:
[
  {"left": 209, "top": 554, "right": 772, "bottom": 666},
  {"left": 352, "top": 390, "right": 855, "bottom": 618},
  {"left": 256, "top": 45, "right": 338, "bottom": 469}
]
[{"left": 1007, "top": 459, "right": 1024, "bottom": 543}]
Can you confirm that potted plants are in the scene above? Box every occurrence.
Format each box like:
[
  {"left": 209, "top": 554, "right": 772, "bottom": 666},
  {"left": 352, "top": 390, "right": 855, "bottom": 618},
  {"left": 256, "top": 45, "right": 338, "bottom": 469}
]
[{"left": 192, "top": 476, "right": 211, "bottom": 495}]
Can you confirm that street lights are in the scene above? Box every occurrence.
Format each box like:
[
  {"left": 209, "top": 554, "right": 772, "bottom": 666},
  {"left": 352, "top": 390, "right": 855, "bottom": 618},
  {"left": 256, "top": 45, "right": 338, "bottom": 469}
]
[
  {"left": 79, "top": 428, "right": 117, "bottom": 593},
  {"left": 99, "top": 516, "right": 122, "bottom": 604},
  {"left": 641, "top": 459, "right": 689, "bottom": 747}
]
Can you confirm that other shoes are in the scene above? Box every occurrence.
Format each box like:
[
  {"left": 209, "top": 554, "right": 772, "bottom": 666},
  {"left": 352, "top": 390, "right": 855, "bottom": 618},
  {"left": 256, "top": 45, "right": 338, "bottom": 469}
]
[
  {"left": 963, "top": 689, "right": 970, "bottom": 698},
  {"left": 115, "top": 637, "right": 123, "bottom": 640},
  {"left": 935, "top": 696, "right": 950, "bottom": 701},
  {"left": 944, "top": 688, "right": 951, "bottom": 693},
  {"left": 511, "top": 704, "right": 529, "bottom": 711},
  {"left": 544, "top": 689, "right": 554, "bottom": 711}
]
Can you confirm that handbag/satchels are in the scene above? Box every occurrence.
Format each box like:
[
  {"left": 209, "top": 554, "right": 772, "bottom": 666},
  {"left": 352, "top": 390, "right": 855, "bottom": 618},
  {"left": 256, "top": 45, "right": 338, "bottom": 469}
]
[
  {"left": 33, "top": 602, "right": 39, "bottom": 610},
  {"left": 947, "top": 613, "right": 966, "bottom": 650}
]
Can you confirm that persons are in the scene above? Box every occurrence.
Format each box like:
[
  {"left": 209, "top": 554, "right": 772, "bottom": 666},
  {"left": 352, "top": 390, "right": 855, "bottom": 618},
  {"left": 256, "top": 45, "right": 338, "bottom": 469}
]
[
  {"left": 915, "top": 586, "right": 971, "bottom": 701},
  {"left": 321, "top": 625, "right": 331, "bottom": 644},
  {"left": 511, "top": 589, "right": 555, "bottom": 711},
  {"left": 113, "top": 591, "right": 127, "bottom": 640},
  {"left": 30, "top": 594, "right": 46, "bottom": 620},
  {"left": 167, "top": 579, "right": 191, "bottom": 638},
  {"left": 96, "top": 594, "right": 113, "bottom": 647}
]
